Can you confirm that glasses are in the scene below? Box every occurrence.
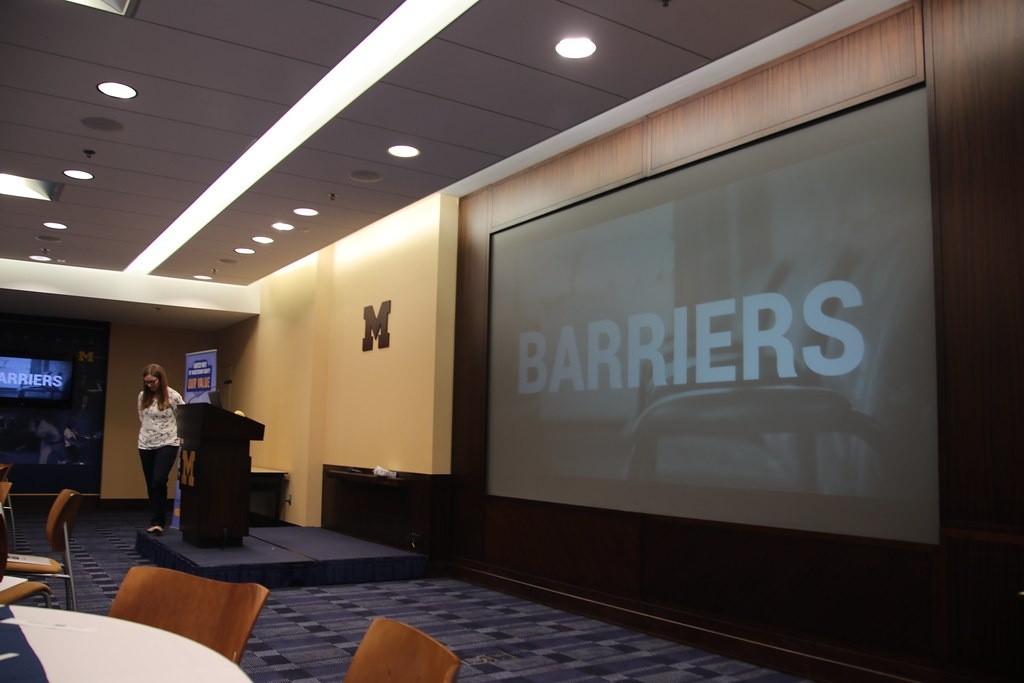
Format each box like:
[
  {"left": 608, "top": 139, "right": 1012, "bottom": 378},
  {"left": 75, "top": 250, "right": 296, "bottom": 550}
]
[{"left": 146, "top": 379, "right": 157, "bottom": 383}]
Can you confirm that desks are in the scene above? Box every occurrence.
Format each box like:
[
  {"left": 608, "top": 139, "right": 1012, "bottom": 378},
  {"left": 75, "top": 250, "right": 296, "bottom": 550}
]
[
  {"left": 250, "top": 467, "right": 288, "bottom": 521},
  {"left": 0, "top": 603, "right": 252, "bottom": 683}
]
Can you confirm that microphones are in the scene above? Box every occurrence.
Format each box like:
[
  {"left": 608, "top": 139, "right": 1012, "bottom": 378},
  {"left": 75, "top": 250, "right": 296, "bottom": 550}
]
[
  {"left": 188, "top": 378, "right": 234, "bottom": 403},
  {"left": 347, "top": 468, "right": 363, "bottom": 473}
]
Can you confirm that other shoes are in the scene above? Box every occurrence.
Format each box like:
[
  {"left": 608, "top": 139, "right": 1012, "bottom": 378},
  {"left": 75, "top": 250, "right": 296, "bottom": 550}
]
[
  {"left": 148, "top": 527, "right": 152, "bottom": 532},
  {"left": 152, "top": 525, "right": 163, "bottom": 532}
]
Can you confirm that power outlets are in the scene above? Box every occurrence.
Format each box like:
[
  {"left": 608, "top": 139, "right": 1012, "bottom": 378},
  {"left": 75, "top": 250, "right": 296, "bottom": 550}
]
[{"left": 285, "top": 495, "right": 293, "bottom": 506}]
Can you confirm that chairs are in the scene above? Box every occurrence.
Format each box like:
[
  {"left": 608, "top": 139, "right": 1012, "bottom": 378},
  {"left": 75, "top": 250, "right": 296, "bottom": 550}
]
[
  {"left": 340, "top": 614, "right": 463, "bottom": 683},
  {"left": 0, "top": 462, "right": 82, "bottom": 612},
  {"left": 108, "top": 564, "right": 271, "bottom": 666}
]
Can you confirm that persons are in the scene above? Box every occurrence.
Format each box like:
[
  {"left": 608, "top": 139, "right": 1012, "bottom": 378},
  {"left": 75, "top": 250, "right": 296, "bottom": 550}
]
[
  {"left": 138, "top": 364, "right": 185, "bottom": 533},
  {"left": 63, "top": 421, "right": 87, "bottom": 458}
]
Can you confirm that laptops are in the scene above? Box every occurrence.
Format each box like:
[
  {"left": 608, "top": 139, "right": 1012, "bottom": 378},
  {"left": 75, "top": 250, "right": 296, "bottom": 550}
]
[{"left": 208, "top": 390, "right": 222, "bottom": 409}]
